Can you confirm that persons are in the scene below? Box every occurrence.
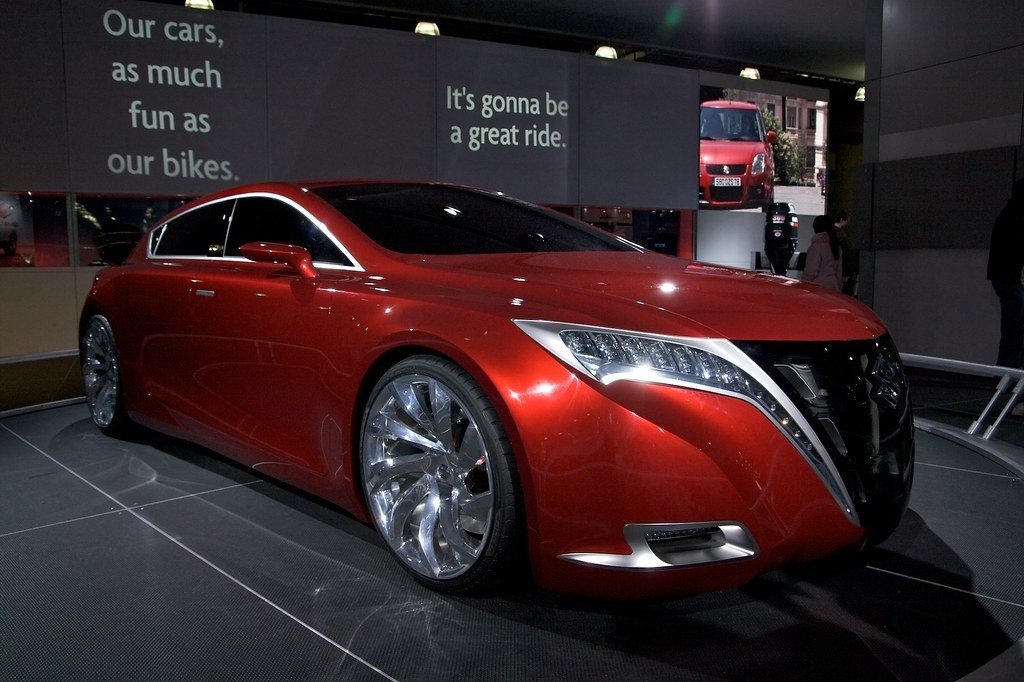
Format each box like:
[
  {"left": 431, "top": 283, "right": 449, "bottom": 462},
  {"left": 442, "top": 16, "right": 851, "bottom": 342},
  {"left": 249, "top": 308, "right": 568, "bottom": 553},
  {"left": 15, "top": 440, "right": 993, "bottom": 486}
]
[
  {"left": 800, "top": 204, "right": 864, "bottom": 291},
  {"left": 986, "top": 175, "right": 1024, "bottom": 418}
]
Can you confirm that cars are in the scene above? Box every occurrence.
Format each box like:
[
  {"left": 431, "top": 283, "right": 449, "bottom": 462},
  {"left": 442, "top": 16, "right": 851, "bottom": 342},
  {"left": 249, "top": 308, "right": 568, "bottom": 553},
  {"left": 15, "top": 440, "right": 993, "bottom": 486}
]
[
  {"left": 0, "top": 192, "right": 114, "bottom": 266},
  {"left": 75, "top": 182, "right": 920, "bottom": 597},
  {"left": 698, "top": 99, "right": 775, "bottom": 209}
]
